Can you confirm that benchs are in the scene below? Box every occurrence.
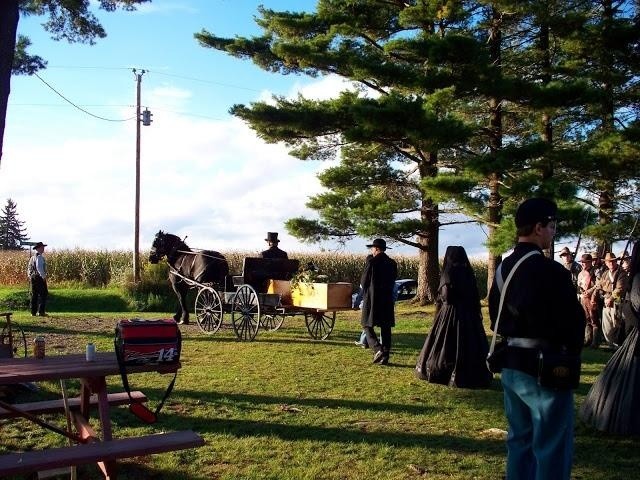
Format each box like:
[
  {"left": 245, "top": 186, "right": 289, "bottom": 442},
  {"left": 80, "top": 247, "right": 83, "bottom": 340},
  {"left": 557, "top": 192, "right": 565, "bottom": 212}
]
[
  {"left": 1, "top": 386, "right": 148, "bottom": 425},
  {"left": 0, "top": 423, "right": 207, "bottom": 480}
]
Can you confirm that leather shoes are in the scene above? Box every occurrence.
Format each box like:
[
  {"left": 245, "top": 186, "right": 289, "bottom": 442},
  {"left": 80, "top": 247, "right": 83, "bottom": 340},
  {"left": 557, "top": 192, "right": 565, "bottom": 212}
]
[
  {"left": 354, "top": 341, "right": 367, "bottom": 349},
  {"left": 373, "top": 350, "right": 385, "bottom": 364},
  {"left": 381, "top": 354, "right": 389, "bottom": 365}
]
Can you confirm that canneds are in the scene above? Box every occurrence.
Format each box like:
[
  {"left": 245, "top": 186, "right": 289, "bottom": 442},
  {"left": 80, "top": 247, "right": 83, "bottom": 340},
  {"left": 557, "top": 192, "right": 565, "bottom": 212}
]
[{"left": 34, "top": 337, "right": 44, "bottom": 360}]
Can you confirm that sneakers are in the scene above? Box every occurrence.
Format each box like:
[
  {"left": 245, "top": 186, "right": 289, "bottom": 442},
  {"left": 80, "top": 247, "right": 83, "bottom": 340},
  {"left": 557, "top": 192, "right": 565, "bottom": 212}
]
[
  {"left": 37, "top": 312, "right": 49, "bottom": 317},
  {"left": 30, "top": 313, "right": 36, "bottom": 317}
]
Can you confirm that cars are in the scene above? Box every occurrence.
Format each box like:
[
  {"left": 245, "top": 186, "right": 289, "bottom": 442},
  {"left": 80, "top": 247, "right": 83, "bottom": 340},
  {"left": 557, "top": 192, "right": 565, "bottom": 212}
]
[{"left": 352, "top": 279, "right": 418, "bottom": 310}]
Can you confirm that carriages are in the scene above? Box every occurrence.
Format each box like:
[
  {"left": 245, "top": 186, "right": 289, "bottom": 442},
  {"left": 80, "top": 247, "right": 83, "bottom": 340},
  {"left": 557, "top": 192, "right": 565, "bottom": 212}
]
[{"left": 148, "top": 229, "right": 336, "bottom": 342}]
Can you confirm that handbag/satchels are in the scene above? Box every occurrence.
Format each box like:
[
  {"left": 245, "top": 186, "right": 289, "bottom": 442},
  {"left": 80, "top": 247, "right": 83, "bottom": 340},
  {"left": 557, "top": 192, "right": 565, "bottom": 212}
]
[
  {"left": 114, "top": 317, "right": 181, "bottom": 376},
  {"left": 484, "top": 341, "right": 507, "bottom": 375}
]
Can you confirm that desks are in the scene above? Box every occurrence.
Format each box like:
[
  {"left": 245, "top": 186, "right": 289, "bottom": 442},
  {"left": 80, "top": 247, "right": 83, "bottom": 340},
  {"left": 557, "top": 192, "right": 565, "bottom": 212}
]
[{"left": 0, "top": 348, "right": 180, "bottom": 480}]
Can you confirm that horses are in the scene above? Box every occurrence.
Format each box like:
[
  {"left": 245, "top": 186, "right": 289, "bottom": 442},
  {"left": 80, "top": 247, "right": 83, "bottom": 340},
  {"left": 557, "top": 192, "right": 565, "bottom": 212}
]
[{"left": 149, "top": 230, "right": 230, "bottom": 327}]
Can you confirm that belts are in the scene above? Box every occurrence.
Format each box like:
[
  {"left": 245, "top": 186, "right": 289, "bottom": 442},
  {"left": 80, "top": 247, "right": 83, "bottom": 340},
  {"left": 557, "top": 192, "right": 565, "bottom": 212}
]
[{"left": 500, "top": 335, "right": 569, "bottom": 356}]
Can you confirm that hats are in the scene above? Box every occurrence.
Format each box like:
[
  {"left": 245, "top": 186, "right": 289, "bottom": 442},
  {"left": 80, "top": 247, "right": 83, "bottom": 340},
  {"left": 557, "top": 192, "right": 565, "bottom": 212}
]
[
  {"left": 558, "top": 245, "right": 577, "bottom": 257},
  {"left": 513, "top": 197, "right": 566, "bottom": 226},
  {"left": 613, "top": 250, "right": 633, "bottom": 260},
  {"left": 264, "top": 231, "right": 281, "bottom": 243},
  {"left": 600, "top": 252, "right": 620, "bottom": 262},
  {"left": 590, "top": 250, "right": 599, "bottom": 260},
  {"left": 31, "top": 241, "right": 48, "bottom": 250},
  {"left": 577, "top": 253, "right": 593, "bottom": 263},
  {"left": 364, "top": 238, "right": 393, "bottom": 251}
]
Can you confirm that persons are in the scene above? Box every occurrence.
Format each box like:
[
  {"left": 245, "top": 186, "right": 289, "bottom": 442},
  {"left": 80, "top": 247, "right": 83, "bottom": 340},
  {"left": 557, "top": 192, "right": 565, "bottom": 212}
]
[
  {"left": 259, "top": 241, "right": 288, "bottom": 258},
  {"left": 416, "top": 246, "right": 493, "bottom": 388},
  {"left": 559, "top": 241, "right": 640, "bottom": 435},
  {"left": 361, "top": 239, "right": 397, "bottom": 365},
  {"left": 489, "top": 199, "right": 586, "bottom": 480},
  {"left": 355, "top": 255, "right": 383, "bottom": 349},
  {"left": 28, "top": 242, "right": 48, "bottom": 316}
]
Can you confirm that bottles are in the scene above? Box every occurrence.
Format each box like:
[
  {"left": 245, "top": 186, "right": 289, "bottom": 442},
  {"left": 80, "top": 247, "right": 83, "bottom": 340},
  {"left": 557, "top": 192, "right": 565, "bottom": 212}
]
[{"left": 34, "top": 336, "right": 46, "bottom": 359}]
